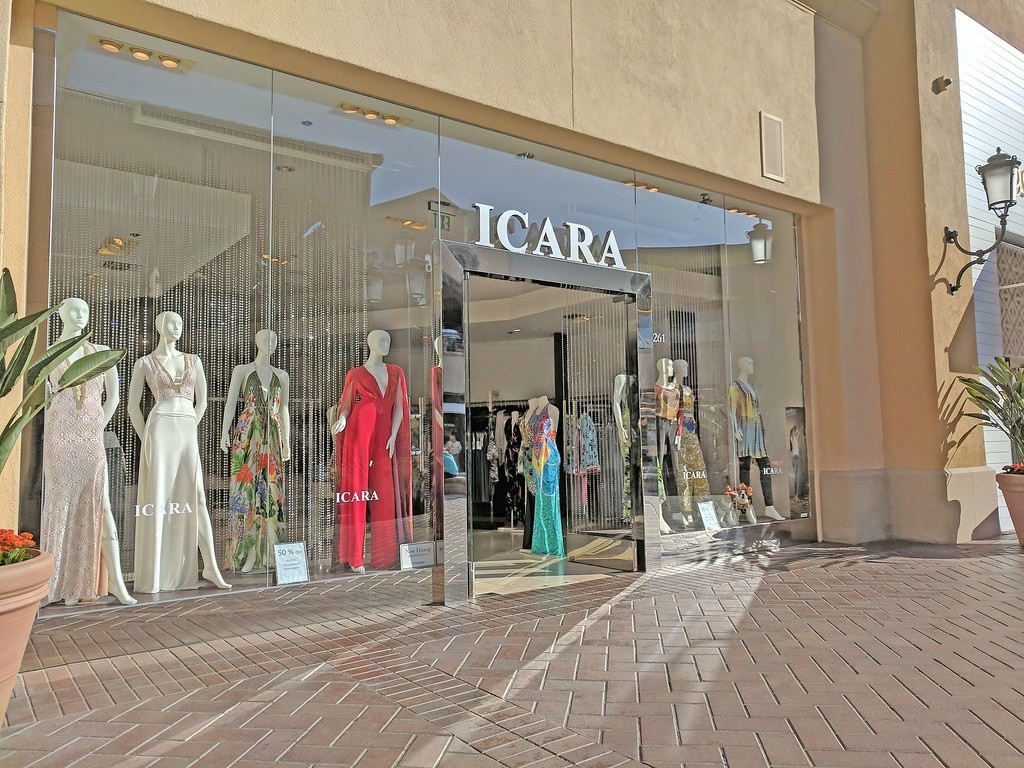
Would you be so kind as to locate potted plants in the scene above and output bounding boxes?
[0,267,127,716]
[957,355,1024,551]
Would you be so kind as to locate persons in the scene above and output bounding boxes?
[504,396,565,558]
[332,330,413,572]
[790,425,801,503]
[40,297,138,605]
[728,357,786,523]
[129,310,233,594]
[221,329,290,573]
[613,369,676,534]
[447,435,461,472]
[655,356,711,527]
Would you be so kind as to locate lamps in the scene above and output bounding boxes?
[945,147,1024,296]
[746,217,773,264]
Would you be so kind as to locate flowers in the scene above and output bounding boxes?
[0,529,37,566]
